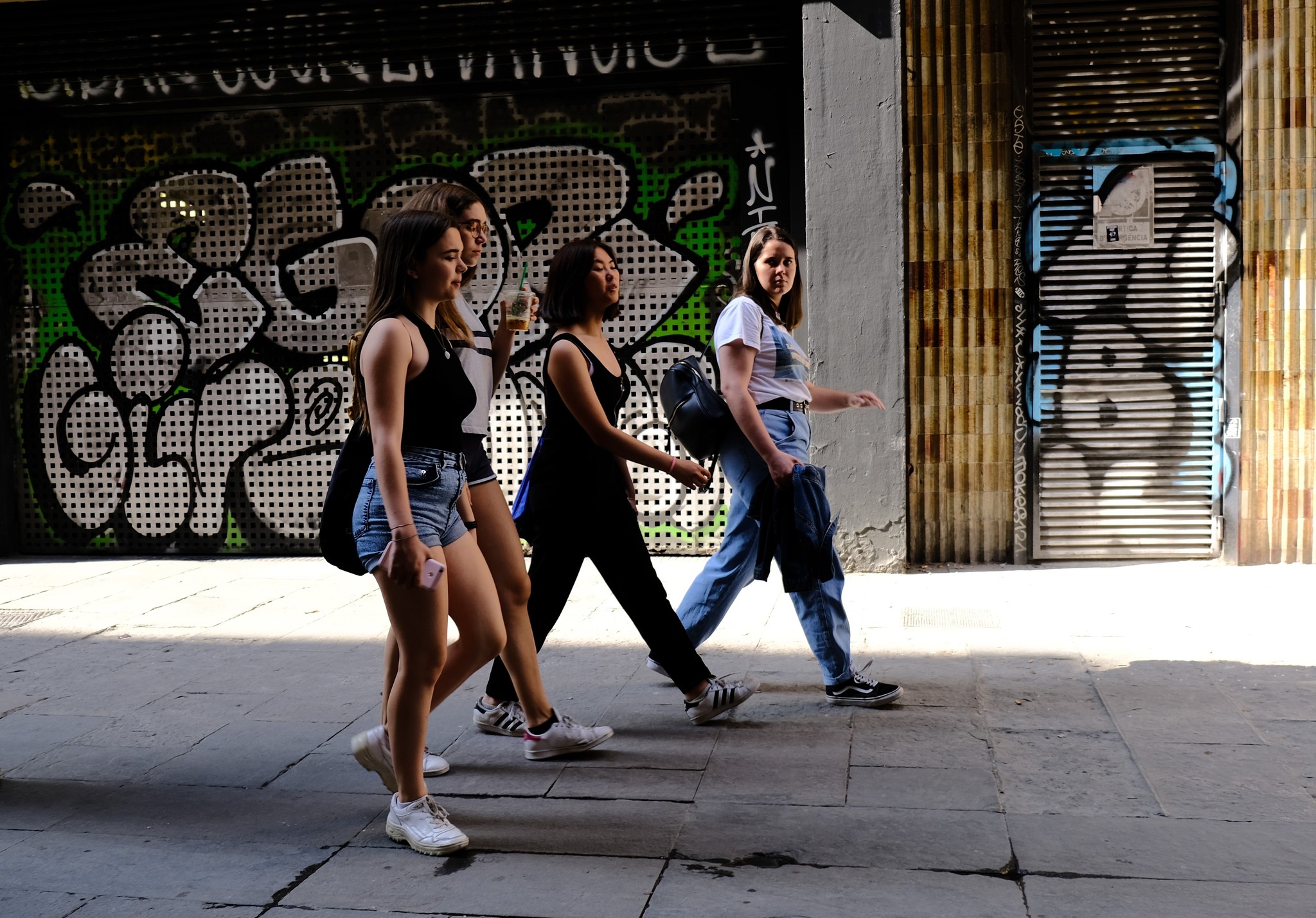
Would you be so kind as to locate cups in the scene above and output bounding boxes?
[500,290,536,331]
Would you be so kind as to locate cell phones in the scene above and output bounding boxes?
[377,541,446,592]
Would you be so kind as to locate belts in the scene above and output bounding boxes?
[756,398,810,415]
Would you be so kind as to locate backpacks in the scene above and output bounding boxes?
[658,354,743,494]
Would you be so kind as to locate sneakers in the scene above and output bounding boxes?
[523,706,614,759]
[421,746,450,777]
[645,650,717,682]
[826,657,904,707]
[472,695,528,737]
[350,725,397,794]
[385,792,469,856]
[683,671,760,725]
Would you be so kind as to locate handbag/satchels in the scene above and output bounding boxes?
[508,429,544,546]
[318,415,374,576]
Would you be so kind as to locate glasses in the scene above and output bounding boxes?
[456,221,489,239]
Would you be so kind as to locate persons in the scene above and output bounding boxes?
[352,211,509,855]
[472,239,762,736]
[646,226,904,708]
[380,184,615,776]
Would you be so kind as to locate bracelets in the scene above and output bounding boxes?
[626,496,636,500]
[665,456,677,475]
[392,533,418,541]
[462,521,478,529]
[390,523,415,531]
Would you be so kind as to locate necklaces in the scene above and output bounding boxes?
[407,306,450,359]
